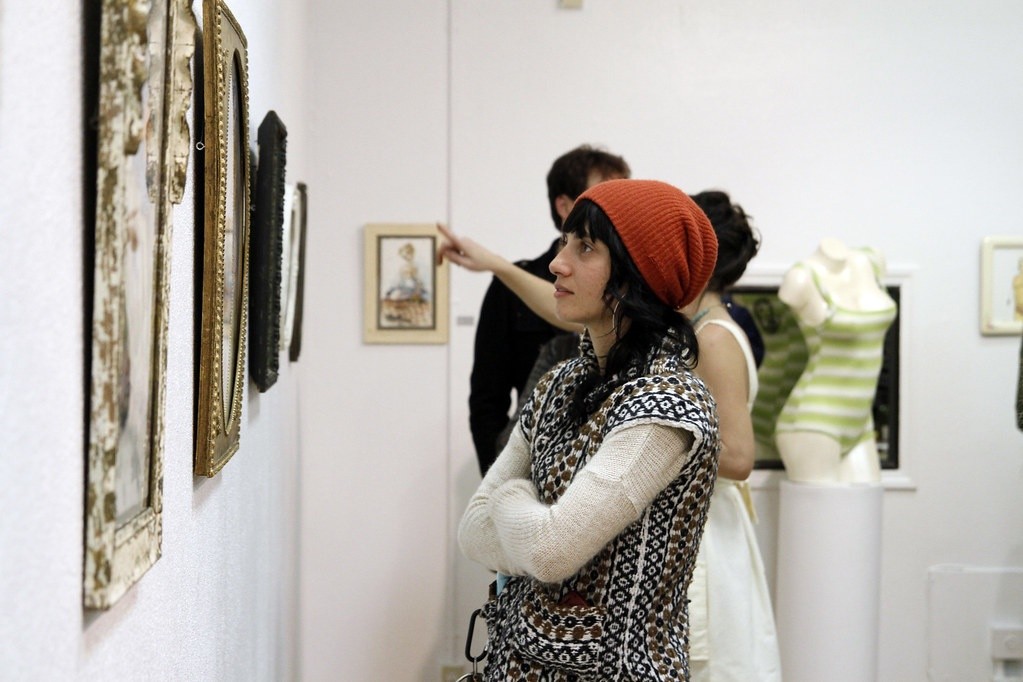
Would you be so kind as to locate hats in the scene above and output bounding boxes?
[577,179,720,310]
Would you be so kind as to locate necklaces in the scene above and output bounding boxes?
[690,299,722,327]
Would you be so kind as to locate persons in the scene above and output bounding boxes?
[467,145,635,480]
[777,243,896,484]
[457,179,721,682]
[436,189,780,682]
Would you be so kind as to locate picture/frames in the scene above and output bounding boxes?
[979,236,1023,337]
[74,0,195,611]
[362,224,451,345]
[249,106,287,392]
[724,265,920,491]
[193,0,250,478]
[287,181,306,363]
